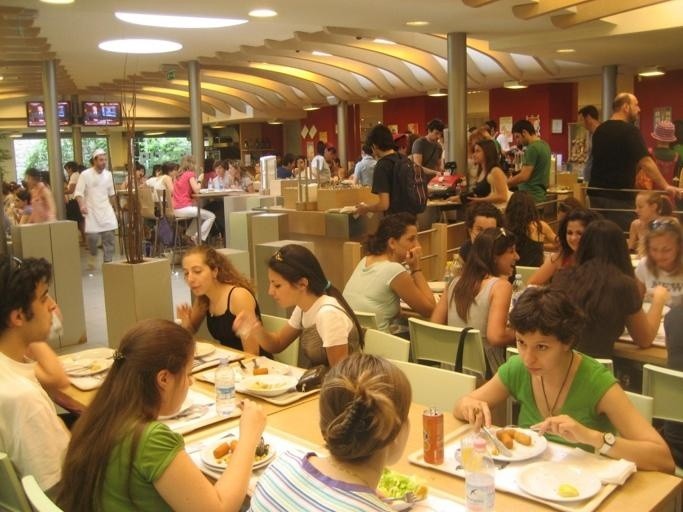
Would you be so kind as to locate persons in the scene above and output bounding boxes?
[578,92,682,229]
[2,144,336,264]
[452,286,675,476]
[173,243,365,372]
[329,117,551,228]
[53,318,266,512]
[342,190,681,396]
[0,255,73,512]
[243,352,413,512]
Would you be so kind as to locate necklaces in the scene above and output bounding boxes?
[540,350,573,417]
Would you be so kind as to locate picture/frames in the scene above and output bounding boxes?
[551,118,563,134]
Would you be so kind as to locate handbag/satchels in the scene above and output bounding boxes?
[636,167,653,190]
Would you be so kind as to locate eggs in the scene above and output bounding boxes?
[555,483,581,498]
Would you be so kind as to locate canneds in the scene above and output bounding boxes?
[423,407,444,464]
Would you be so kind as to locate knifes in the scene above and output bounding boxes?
[482,425,513,458]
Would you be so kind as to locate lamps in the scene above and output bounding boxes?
[503,80,529,90]
[209,88,447,130]
[637,66,668,76]
[7,128,167,140]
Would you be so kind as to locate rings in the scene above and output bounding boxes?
[473,407,480,415]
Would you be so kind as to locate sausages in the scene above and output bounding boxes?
[230,439,237,453]
[253,368,266,374]
[214,440,230,457]
[496,426,533,449]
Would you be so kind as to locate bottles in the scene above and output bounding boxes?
[511,274,524,308]
[208,177,214,191]
[215,358,235,415]
[465,439,496,512]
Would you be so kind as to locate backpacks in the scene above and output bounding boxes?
[379,152,426,214]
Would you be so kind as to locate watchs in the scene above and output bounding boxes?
[597,433,615,456]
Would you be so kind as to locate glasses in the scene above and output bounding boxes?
[7,252,22,291]
[649,220,675,230]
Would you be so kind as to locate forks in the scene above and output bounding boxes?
[379,492,417,503]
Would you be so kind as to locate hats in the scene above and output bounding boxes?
[92,148,106,158]
[323,142,335,150]
[392,133,408,146]
[650,121,677,142]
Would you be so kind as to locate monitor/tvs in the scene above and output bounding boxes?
[81,101,122,126]
[25,101,72,127]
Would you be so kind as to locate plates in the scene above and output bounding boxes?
[516,462,601,502]
[65,359,114,376]
[201,438,277,472]
[192,342,216,358]
[462,429,548,461]
[239,375,298,396]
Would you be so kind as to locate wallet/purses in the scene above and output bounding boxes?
[296,364,327,391]
